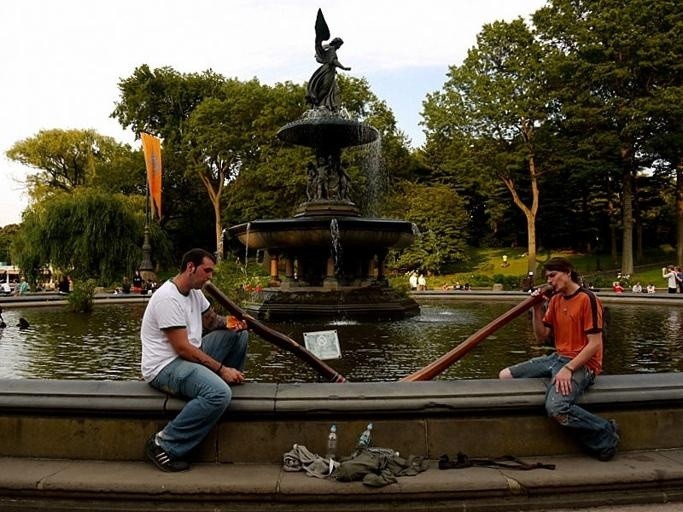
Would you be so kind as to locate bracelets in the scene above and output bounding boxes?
[215,362,222,374]
[563,365,574,372]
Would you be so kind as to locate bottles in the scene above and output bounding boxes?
[356,422,374,451]
[327,424,339,463]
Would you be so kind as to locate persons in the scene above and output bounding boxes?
[521,271,535,293]
[442,281,472,293]
[305,35,351,110]
[408,271,417,291]
[645,283,655,294]
[671,265,682,294]
[612,282,624,294]
[631,281,642,293]
[660,265,677,293]
[0,270,158,299]
[139,248,250,473]
[497,254,622,463]
[417,273,427,291]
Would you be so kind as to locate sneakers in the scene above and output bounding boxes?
[143,433,189,472]
[598,419,620,462]
